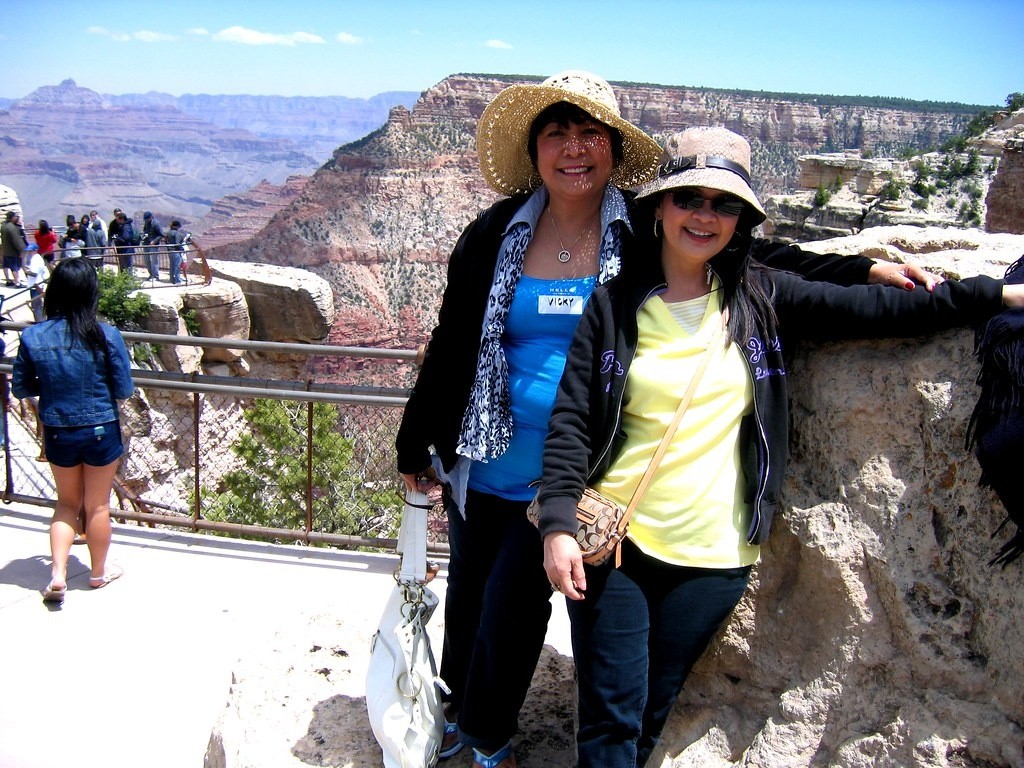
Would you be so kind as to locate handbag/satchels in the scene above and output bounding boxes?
[526,482,631,569]
[365,488,444,768]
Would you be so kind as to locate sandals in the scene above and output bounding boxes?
[43,576,68,602]
[89,563,123,589]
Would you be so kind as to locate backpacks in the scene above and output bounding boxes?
[131,230,141,246]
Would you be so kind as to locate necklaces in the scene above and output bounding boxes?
[547,207,599,263]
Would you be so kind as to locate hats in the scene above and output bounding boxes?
[172,221,182,227]
[25,243,39,251]
[631,126,767,232]
[476,70,665,198]
[143,212,152,221]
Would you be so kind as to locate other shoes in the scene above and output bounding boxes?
[145,276,159,281]
[6,280,28,288]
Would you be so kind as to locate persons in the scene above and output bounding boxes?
[12,256,135,603]
[395,70,947,767]
[532,125,1024,768]
[0,208,190,323]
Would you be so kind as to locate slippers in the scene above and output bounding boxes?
[437,709,514,768]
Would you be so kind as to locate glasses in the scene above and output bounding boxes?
[395,471,452,513]
[666,187,746,217]
